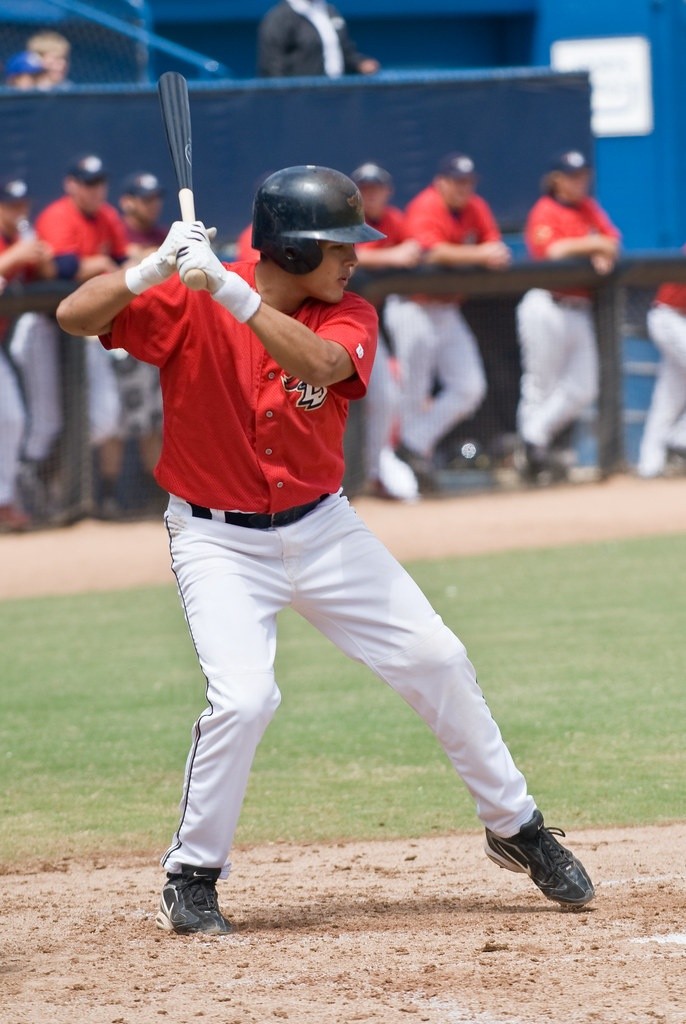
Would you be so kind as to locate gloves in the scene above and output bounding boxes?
[125,221,218,295]
[175,241,262,324]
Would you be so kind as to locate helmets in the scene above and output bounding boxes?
[250,165,387,274]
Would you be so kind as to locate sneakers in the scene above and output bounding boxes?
[484,809,594,907]
[157,865,233,935]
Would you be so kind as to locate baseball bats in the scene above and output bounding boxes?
[158,71,208,290]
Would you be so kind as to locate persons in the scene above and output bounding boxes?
[0,154,170,527]
[236,223,261,262]
[635,285,686,478]
[379,154,512,497]
[491,149,620,492]
[260,0,379,80]
[0,30,73,93]
[57,165,595,934]
[339,162,423,498]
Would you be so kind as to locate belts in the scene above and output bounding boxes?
[187,491,329,530]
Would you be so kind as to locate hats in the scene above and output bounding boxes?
[439,151,481,179]
[548,146,592,173]
[351,163,392,187]
[66,152,109,184]
[0,177,31,201]
[117,171,165,200]
